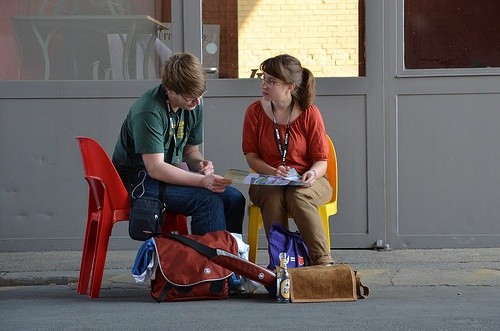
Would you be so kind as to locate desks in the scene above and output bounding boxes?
[14,15,170,80]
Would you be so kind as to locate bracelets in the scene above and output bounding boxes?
[311,168,318,179]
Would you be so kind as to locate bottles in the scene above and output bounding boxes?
[277,253,291,303]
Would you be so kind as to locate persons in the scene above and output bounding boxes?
[242,54,336,267]
[112,51,246,236]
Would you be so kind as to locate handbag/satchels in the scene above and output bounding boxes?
[261,223,311,293]
[274,263,370,304]
[129,197,166,241]
[151,231,239,301]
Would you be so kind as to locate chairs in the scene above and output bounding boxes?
[75,136,188,299]
[248,133,338,264]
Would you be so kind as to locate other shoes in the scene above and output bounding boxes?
[230,285,245,294]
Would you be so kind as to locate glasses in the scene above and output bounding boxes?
[263,76,293,85]
[173,87,208,105]
